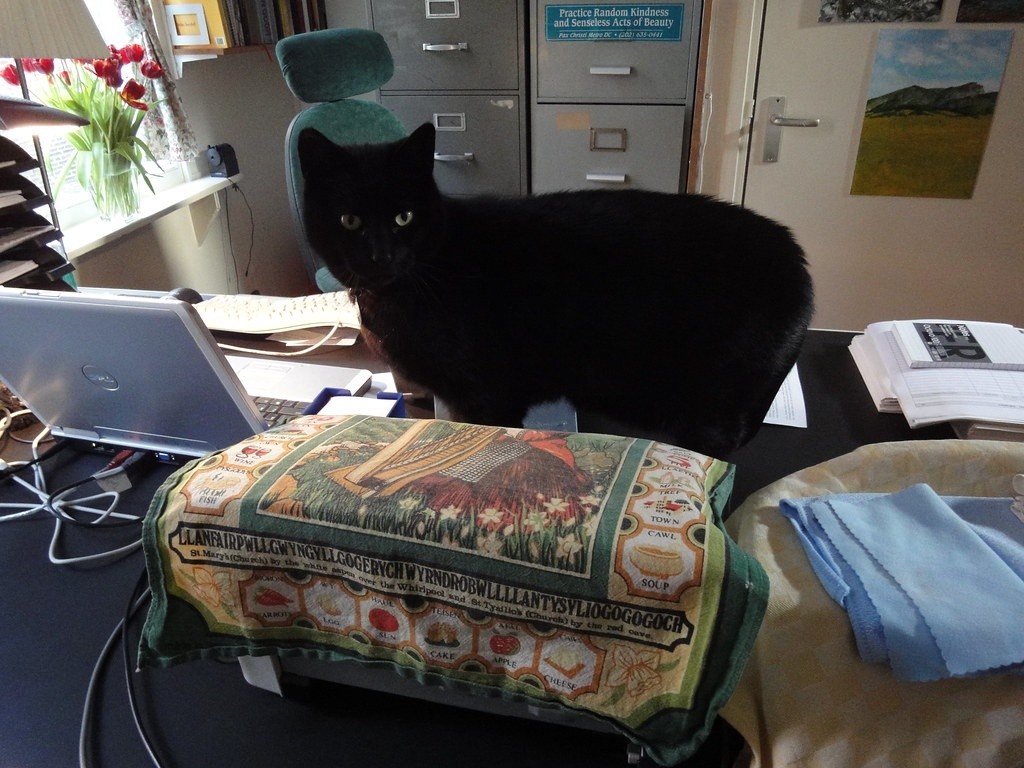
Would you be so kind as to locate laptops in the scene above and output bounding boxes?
[0,286,373,467]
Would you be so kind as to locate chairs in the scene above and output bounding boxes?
[275,27,410,294]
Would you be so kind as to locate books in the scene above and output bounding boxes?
[163,0,328,49]
[849,319,1024,429]
[0,161,55,283]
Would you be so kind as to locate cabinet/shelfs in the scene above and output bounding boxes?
[0,135,77,292]
[530,0,702,198]
[366,0,530,196]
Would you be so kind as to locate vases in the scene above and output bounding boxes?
[88,146,141,224]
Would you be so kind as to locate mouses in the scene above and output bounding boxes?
[161,287,204,305]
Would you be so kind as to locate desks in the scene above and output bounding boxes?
[0,287,958,768]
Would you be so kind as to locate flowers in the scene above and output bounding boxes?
[0,44,169,216]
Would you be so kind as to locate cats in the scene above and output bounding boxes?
[297,121,816,462]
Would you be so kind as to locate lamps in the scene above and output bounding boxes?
[0,95,90,137]
[0,0,111,263]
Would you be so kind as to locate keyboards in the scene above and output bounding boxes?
[191,292,361,335]
